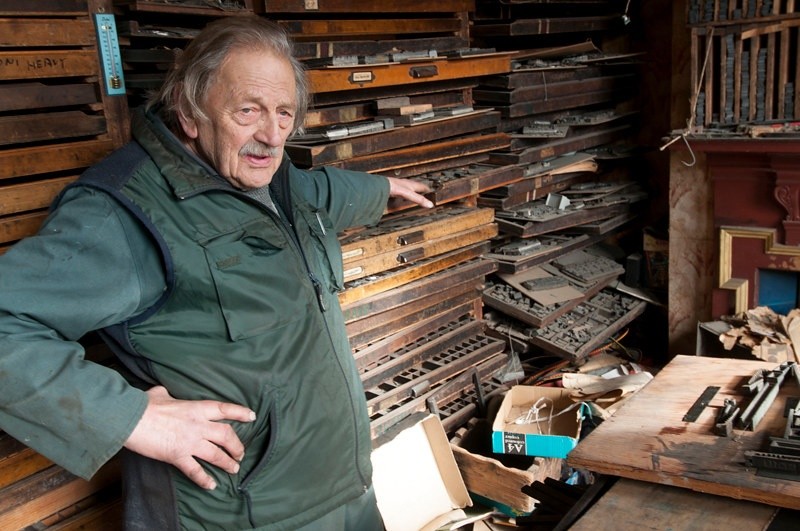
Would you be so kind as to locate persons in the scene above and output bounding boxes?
[0,11,437,531]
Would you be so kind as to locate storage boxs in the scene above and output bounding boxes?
[370,411,474,531]
[492,385,582,459]
[450,419,561,518]
